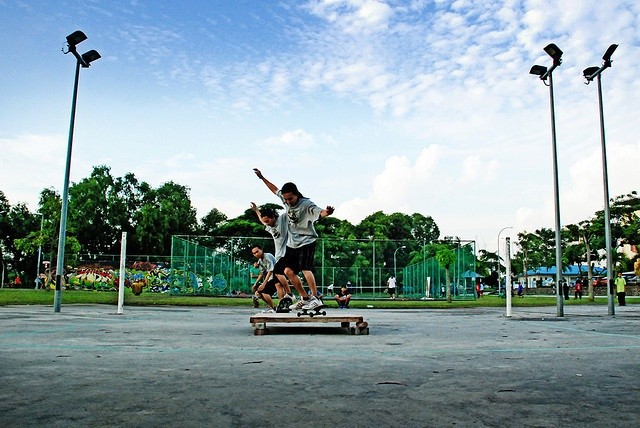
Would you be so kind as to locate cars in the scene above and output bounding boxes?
[600,278,607,286]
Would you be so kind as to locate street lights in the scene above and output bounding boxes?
[34,212,43,289]
[394,246,406,293]
[530,43,563,317]
[54,30,101,311]
[583,44,618,315]
[498,227,513,293]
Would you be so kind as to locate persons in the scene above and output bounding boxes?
[307,285,324,304]
[614,270,627,306]
[582,277,588,287]
[346,280,353,294]
[479,281,484,298]
[475,281,480,298]
[517,282,524,298]
[548,279,556,295]
[250,201,296,301]
[15,275,21,288]
[334,285,352,310]
[327,283,334,297]
[253,167,335,311]
[573,279,583,300]
[250,243,277,313]
[562,279,569,300]
[386,274,398,300]
[249,260,264,309]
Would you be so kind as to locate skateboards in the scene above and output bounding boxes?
[295,304,327,317]
[252,296,259,307]
[276,297,293,312]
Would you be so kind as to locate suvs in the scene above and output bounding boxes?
[592,275,602,287]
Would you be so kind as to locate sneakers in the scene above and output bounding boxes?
[301,294,323,309]
[289,295,310,310]
[284,292,296,301]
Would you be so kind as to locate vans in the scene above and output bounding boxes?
[622,272,637,286]
